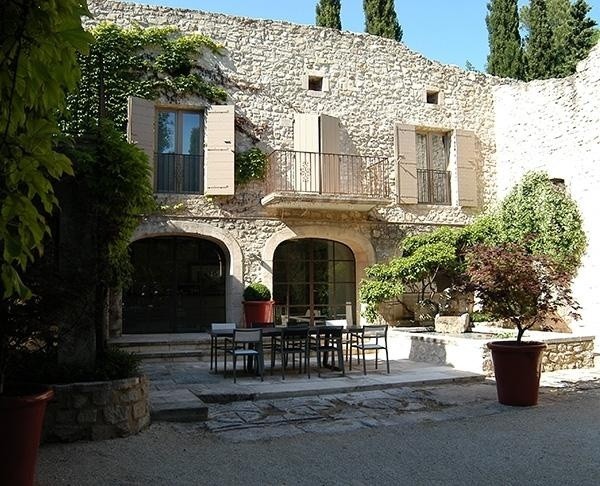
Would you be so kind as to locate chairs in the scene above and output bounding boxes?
[206,319,390,383]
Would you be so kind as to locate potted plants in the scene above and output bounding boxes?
[0,289,96,486]
[458,241,557,406]
[241,282,276,328]
